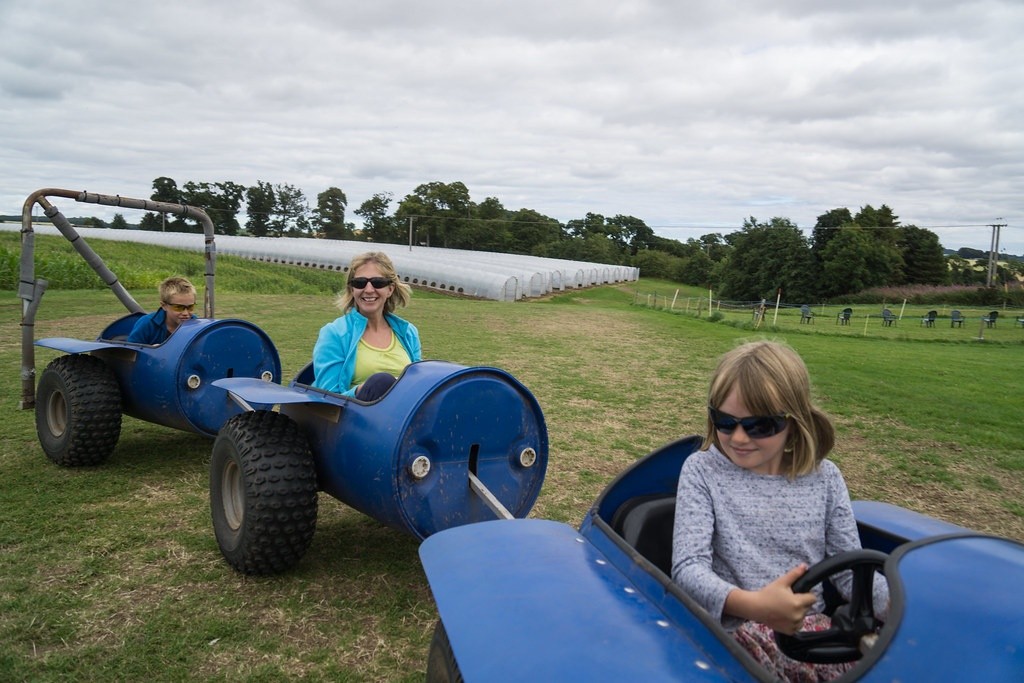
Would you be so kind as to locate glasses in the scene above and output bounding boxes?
[348,277,393,290]
[707,406,792,441]
[163,301,195,313]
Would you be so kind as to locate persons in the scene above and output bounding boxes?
[127,276,199,346]
[310,251,422,401]
[671,341,890,683]
